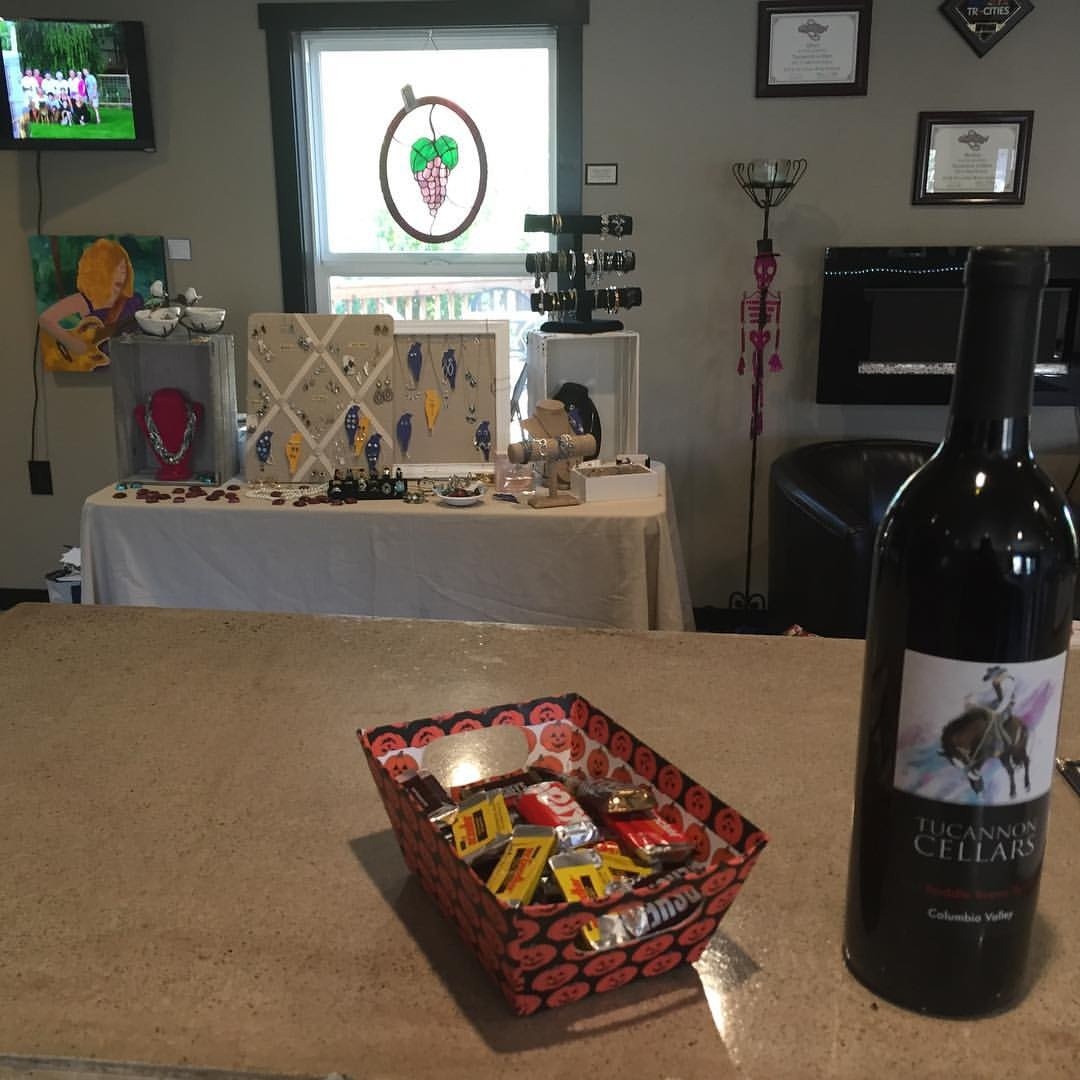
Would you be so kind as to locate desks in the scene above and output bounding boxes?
[0,603,1079,1079]
[81,459,697,632]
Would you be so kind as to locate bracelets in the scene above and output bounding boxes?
[533,214,632,319]
[516,434,575,465]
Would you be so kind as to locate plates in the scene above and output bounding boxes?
[436,490,488,507]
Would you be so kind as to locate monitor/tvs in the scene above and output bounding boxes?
[815,244,1080,407]
[0,17,159,153]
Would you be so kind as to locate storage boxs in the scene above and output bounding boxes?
[108,332,236,487]
[356,691,769,1019]
[526,330,639,461]
[569,463,659,502]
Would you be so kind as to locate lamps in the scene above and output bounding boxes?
[729,157,809,611]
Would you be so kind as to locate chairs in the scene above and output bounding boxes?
[768,439,941,641]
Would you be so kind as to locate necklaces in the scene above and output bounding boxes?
[145,389,195,465]
[396,318,497,425]
[566,396,596,434]
[533,414,576,468]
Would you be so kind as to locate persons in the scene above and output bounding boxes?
[21,69,100,124]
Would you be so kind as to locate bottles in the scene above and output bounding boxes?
[843,247,1079,1016]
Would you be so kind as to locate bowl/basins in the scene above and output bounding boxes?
[134,307,181,336]
[186,306,227,331]
